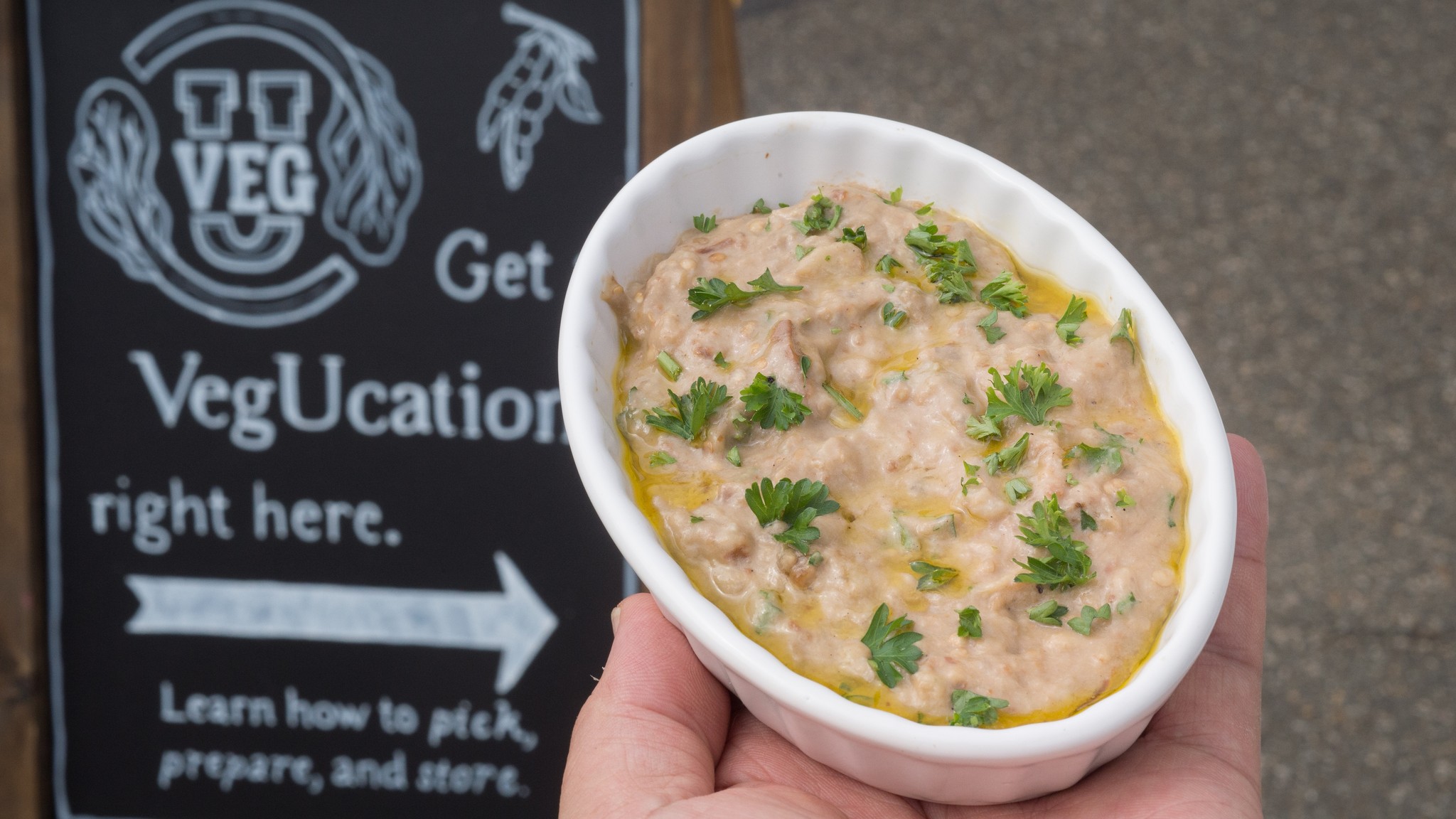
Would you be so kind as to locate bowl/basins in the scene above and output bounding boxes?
[557,110,1237,807]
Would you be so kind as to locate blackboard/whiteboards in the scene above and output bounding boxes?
[0,0,742,818]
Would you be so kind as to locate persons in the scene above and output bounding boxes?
[558,433,1269,819]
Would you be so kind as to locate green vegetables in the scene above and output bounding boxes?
[644,185,1177,729]
